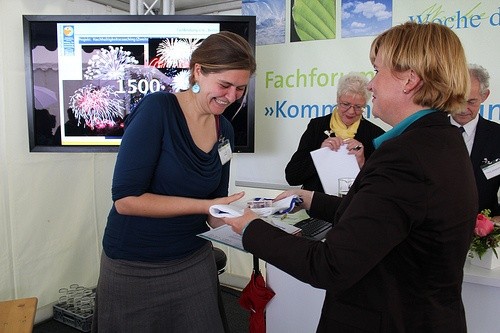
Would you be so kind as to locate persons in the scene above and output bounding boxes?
[284,75,388,195]
[32,106,96,146]
[447,62,500,218]
[91,31,258,333]
[222,19,481,333]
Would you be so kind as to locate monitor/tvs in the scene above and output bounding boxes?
[23,14,255,155]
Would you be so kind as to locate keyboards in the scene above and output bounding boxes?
[293,217,333,240]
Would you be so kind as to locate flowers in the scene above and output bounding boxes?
[468,208,500,259]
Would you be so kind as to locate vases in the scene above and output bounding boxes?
[470,242,500,270]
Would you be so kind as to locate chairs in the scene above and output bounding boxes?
[213,247,230,333]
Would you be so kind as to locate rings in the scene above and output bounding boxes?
[355,145,362,150]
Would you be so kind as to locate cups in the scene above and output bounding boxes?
[57,283,95,317]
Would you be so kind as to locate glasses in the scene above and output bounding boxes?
[339,101,365,111]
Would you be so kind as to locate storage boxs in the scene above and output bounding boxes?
[53,287,97,333]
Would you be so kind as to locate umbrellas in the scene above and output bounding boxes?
[34,85,57,109]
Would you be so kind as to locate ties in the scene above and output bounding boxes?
[459,126,465,134]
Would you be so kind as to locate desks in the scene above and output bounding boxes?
[266,250,500,333]
[0,297,38,333]
